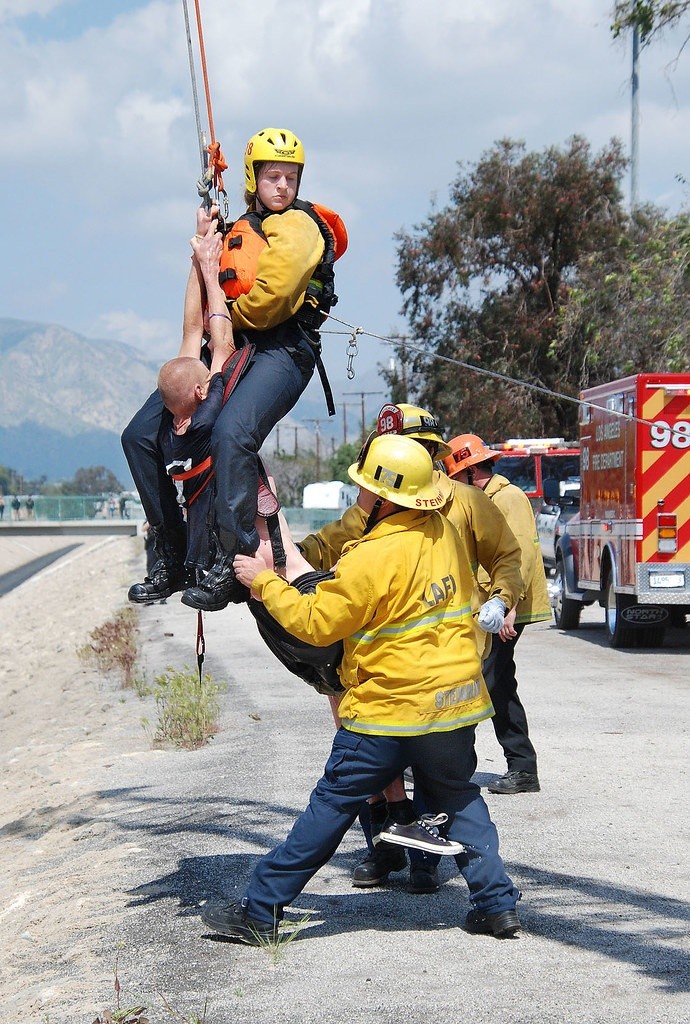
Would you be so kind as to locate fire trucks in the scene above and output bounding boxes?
[489,438,581,515]
[541,373,690,649]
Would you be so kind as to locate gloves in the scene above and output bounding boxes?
[476,597,507,634]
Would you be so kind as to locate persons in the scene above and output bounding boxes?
[12,495,20,521]
[201,434,522,943]
[122,127,348,612]
[158,219,464,856]
[440,434,550,792]
[26,494,35,519]
[109,496,130,520]
[292,402,525,892]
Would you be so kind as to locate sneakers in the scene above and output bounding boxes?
[201,900,279,946]
[409,855,440,893]
[128,557,196,601]
[182,562,254,612]
[352,843,407,886]
[371,823,397,849]
[487,770,539,793]
[465,902,521,936]
[379,815,464,855]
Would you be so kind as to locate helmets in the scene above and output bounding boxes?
[347,432,447,509]
[440,434,502,475]
[381,401,454,461]
[244,128,306,201]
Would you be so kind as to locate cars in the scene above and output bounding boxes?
[535,475,582,577]
